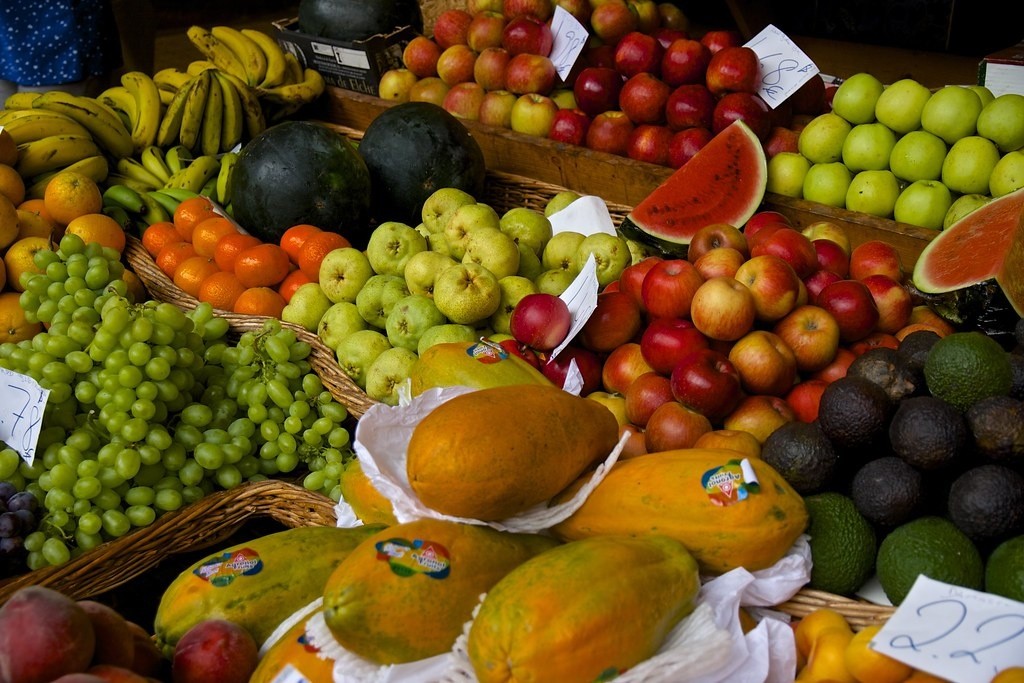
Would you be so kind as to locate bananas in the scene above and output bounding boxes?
[0,25,328,242]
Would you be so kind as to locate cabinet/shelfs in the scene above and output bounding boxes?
[271,0,1024,276]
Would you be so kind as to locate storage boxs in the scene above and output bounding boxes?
[1,473,901,682]
[975,41,1024,100]
[116,119,643,416]
[272,16,435,98]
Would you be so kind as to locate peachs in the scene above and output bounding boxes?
[0,581,258,683]
[738,605,1023,683]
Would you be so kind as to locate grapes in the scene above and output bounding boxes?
[0,234,357,585]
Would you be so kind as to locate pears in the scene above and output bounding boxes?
[282,186,659,409]
[770,72,1023,232]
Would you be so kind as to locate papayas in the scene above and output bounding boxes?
[154,341,808,683]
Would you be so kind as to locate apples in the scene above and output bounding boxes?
[378,0,840,168]
[495,212,956,460]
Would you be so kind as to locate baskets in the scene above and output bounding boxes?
[1,120,904,632]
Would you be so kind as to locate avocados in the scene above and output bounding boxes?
[759,330,1024,607]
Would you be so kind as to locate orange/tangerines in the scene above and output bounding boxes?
[0,126,151,346]
[141,198,352,324]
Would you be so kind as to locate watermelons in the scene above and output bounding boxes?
[617,119,768,261]
[910,188,1024,351]
[229,103,489,246]
[298,0,424,40]
